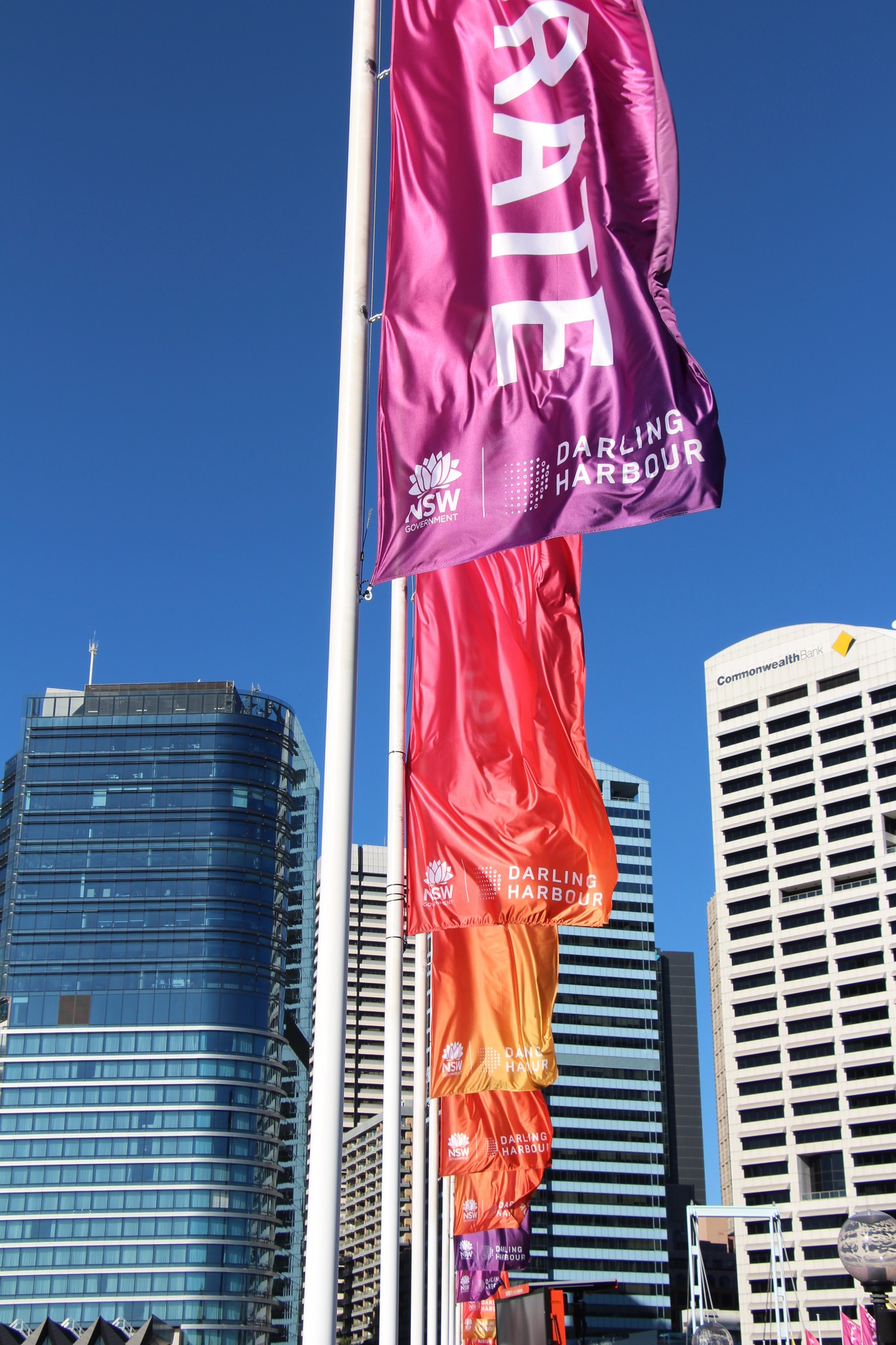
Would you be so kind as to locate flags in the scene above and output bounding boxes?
[453,1168,545,1237]
[406,534,618,935]
[440,1090,554,1177]
[368,0,727,586]
[429,925,560,1101]
[456,1270,506,1303]
[462,1271,510,1345]
[859,1304,878,1345]
[841,1312,862,1345]
[885,1298,896,1310]
[805,1328,820,1345]
[453,1205,532,1272]
